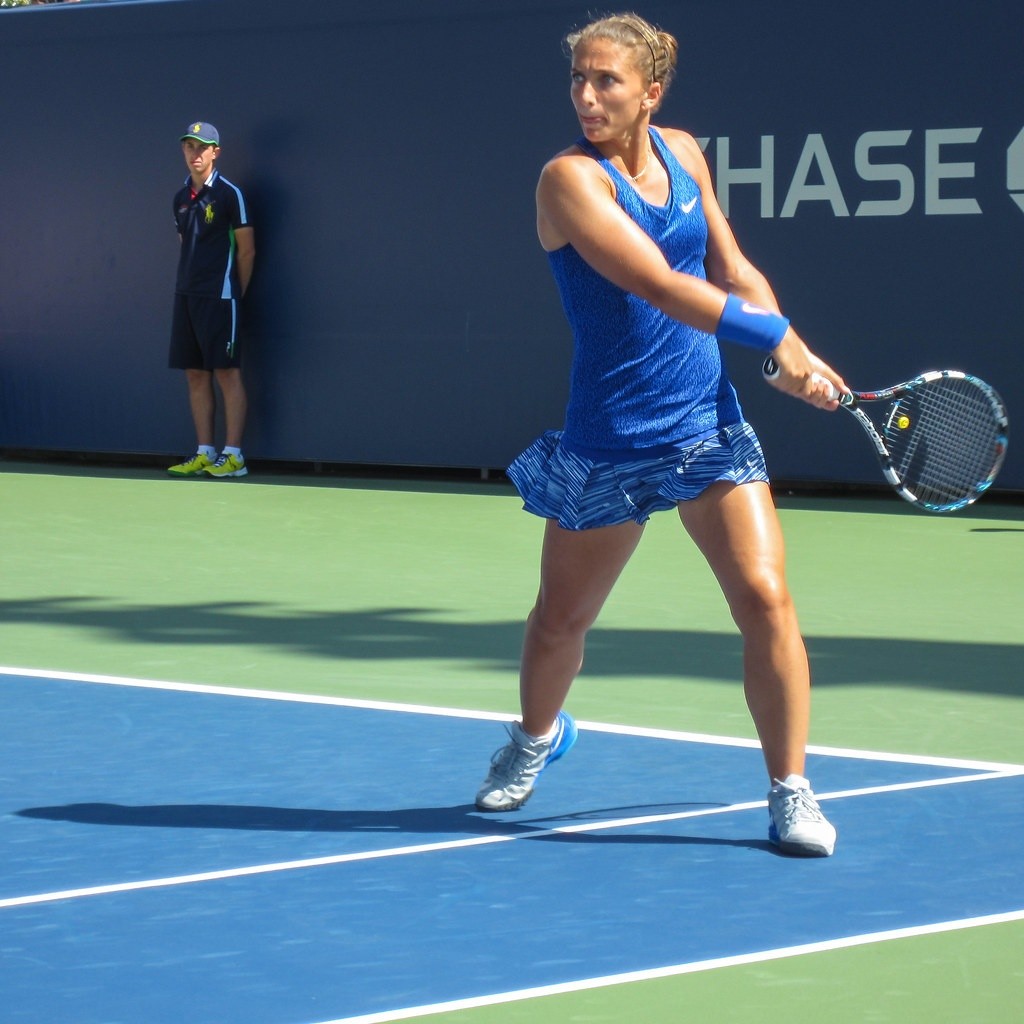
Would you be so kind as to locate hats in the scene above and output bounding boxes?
[178,122,220,146]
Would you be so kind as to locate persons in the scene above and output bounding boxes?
[473,12,853,859]
[162,119,257,480]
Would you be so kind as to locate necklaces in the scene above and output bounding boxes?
[618,132,651,182]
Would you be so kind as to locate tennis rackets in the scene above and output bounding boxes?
[761,355,1010,514]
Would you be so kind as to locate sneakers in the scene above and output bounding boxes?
[202,450,248,477]
[167,451,219,478]
[768,772,836,857]
[474,709,578,810]
